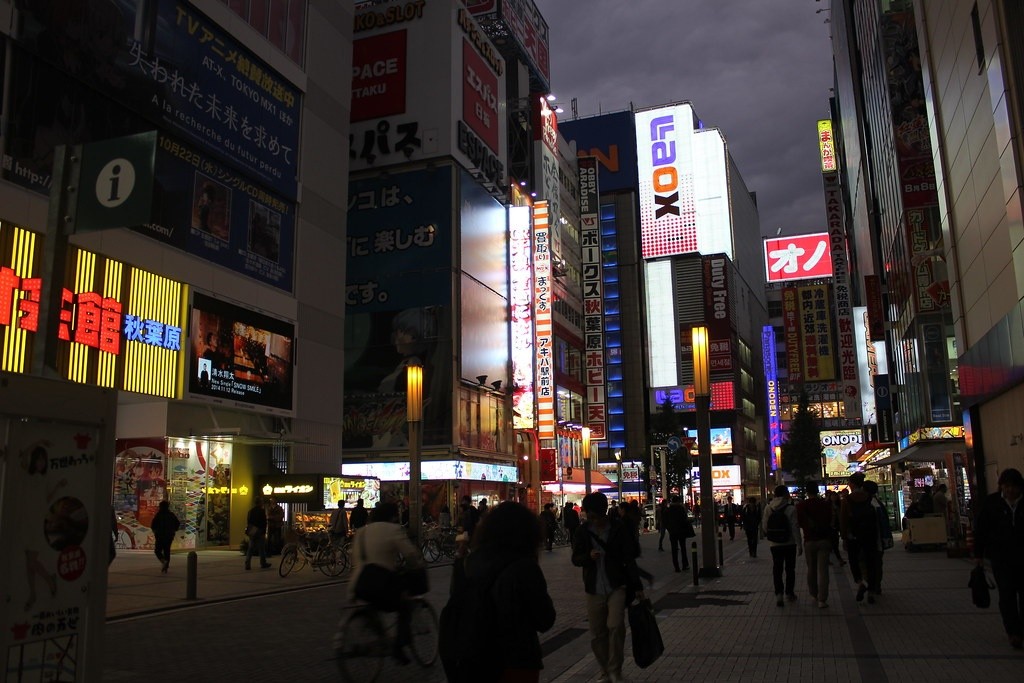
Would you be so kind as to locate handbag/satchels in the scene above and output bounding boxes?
[581,521,644,591]
[245,510,266,541]
[968,561,996,608]
[352,527,404,613]
[332,530,347,538]
[628,597,667,669]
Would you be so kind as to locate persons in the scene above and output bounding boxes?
[243,496,272,570]
[968,466,1024,653]
[571,491,641,683]
[324,471,974,613]
[449,500,557,682]
[150,500,180,573]
[267,495,286,558]
[108,502,118,568]
[344,500,426,668]
[185,175,293,409]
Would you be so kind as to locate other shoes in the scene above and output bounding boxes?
[261,563,272,569]
[675,568,681,572]
[828,562,834,565]
[161,560,171,574]
[245,567,251,570]
[785,590,798,601]
[812,597,818,601]
[818,602,829,608]
[777,601,785,607]
[682,566,690,570]
[544,546,552,552]
[840,560,847,567]
[867,595,877,605]
[856,583,866,602]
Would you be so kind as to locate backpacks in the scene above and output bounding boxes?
[437,551,537,683]
[765,501,792,543]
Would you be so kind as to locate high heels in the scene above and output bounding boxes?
[659,546,665,552]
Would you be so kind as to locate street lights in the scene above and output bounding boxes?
[405,358,425,551]
[582,425,593,498]
[614,448,622,503]
[690,321,725,577]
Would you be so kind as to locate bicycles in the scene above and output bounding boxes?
[421,525,468,562]
[548,521,570,545]
[275,513,355,578]
[324,555,442,683]
